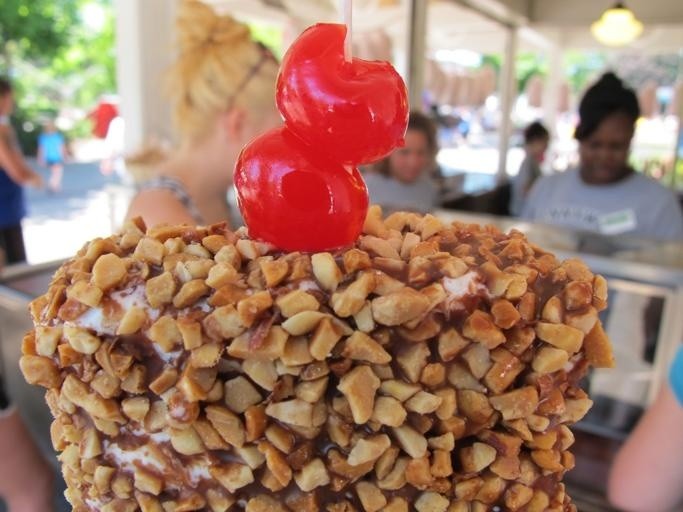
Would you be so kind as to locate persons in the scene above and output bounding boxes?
[0,76,42,266]
[608,344,683,512]
[0,379,57,512]
[37,108,66,169]
[519,72,683,245]
[507,123,549,216]
[379,111,438,185]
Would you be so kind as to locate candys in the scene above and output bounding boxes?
[233,0,409,254]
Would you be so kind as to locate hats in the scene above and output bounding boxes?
[576,73,621,139]
